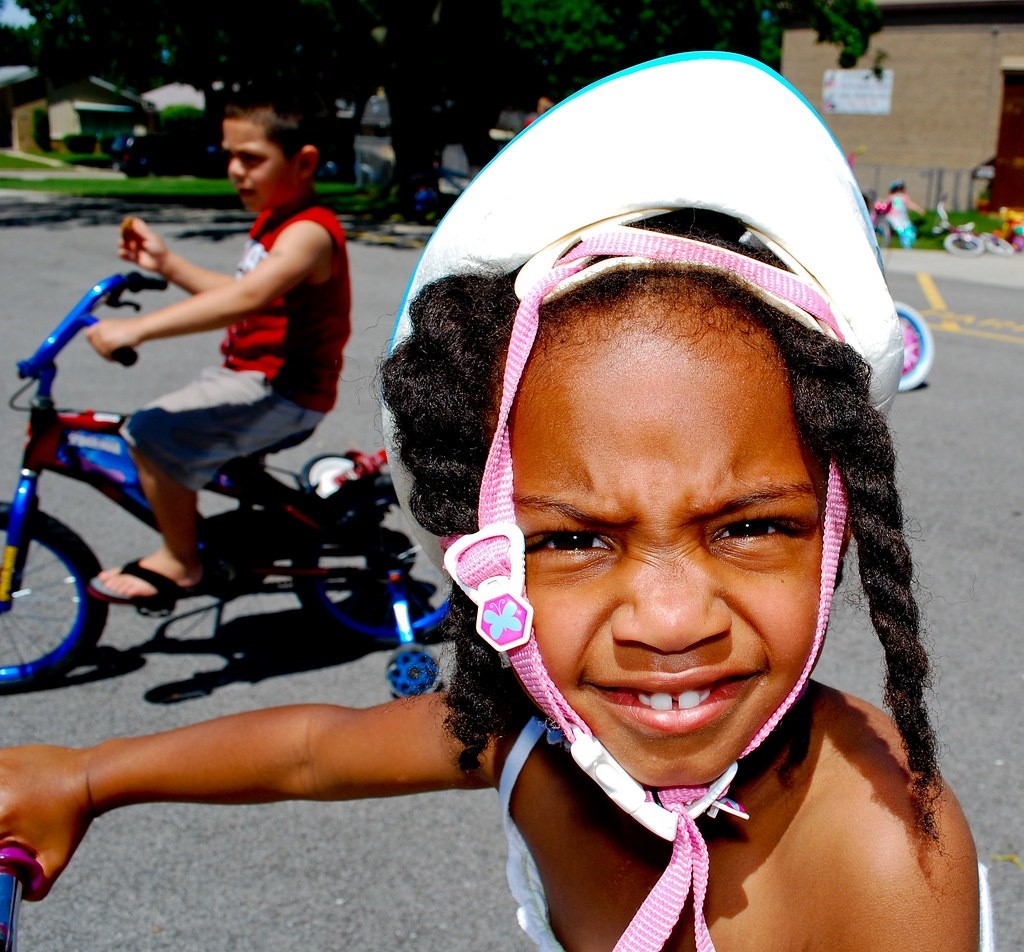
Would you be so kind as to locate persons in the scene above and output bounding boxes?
[0,52,996,952]
[869,181,928,248]
[84,97,356,603]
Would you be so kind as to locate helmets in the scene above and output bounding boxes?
[381,50,905,596]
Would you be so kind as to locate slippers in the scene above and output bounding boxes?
[88,557,218,602]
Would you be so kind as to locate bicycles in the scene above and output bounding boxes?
[887,300,934,393]
[929,194,1014,257]
[0,271,456,696]
